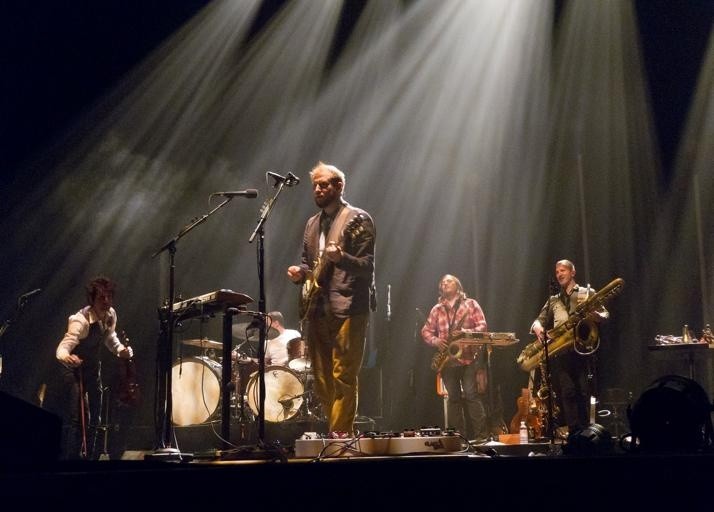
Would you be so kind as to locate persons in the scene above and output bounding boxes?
[530,259,609,431]
[56,278,133,429]
[288,162,375,438]
[422,273,487,436]
[259,310,301,367]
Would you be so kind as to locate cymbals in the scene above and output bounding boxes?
[181,338,223,350]
[231,321,280,341]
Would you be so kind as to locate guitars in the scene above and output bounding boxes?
[511,368,542,439]
[112,329,139,411]
[295,214,365,321]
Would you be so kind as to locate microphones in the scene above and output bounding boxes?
[386,284,391,323]
[22,288,42,299]
[269,171,293,187]
[214,189,257,198]
[289,172,300,185]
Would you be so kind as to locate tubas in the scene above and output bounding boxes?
[517,278,626,372]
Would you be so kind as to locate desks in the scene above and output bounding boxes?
[646,340,714,382]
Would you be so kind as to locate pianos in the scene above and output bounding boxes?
[163,289,253,310]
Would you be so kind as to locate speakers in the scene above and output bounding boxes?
[0,391,63,462]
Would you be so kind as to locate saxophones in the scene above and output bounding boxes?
[431,299,470,372]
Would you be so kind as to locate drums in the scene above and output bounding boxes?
[287,337,313,371]
[165,355,224,426]
[245,365,306,423]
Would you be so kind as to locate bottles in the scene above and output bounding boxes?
[519,421,529,444]
[705,323,712,343]
[683,324,691,344]
